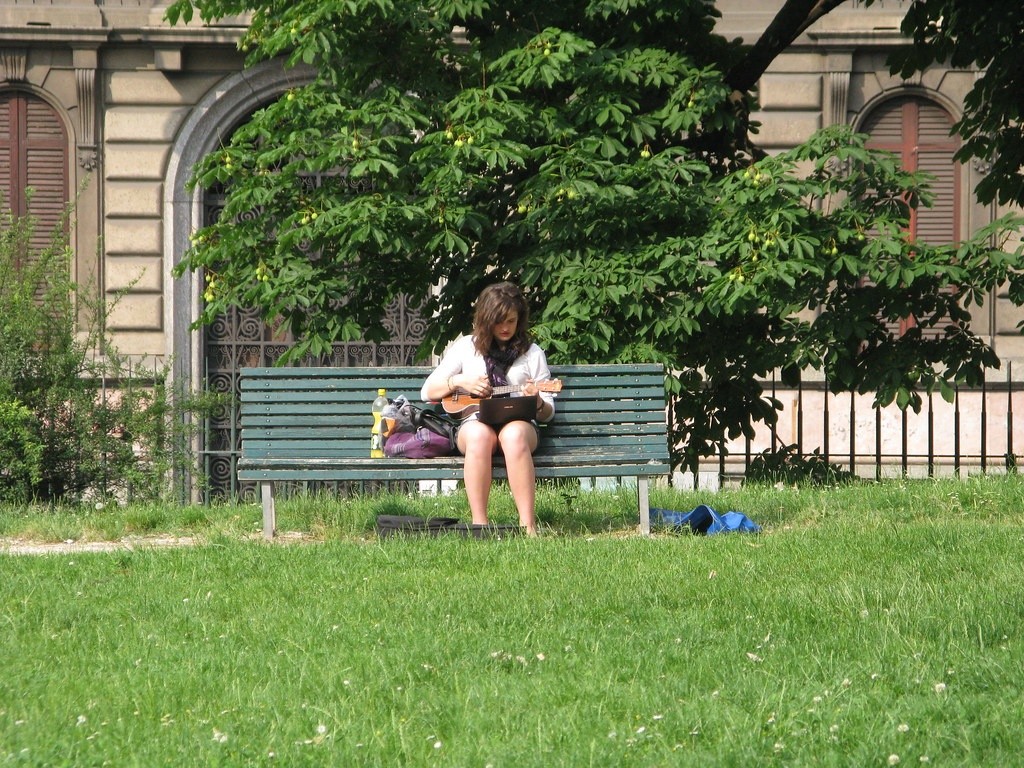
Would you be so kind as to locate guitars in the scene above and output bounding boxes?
[441,376,562,421]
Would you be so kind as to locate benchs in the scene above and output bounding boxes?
[237,362,672,539]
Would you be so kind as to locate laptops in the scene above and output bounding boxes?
[476,395,538,425]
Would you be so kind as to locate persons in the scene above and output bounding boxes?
[421,283,555,537]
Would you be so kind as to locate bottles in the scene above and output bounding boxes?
[370,389,389,458]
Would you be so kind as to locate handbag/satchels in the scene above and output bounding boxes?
[384,408,456,458]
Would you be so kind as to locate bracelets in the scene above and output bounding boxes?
[537,400,545,412]
[447,376,458,391]
